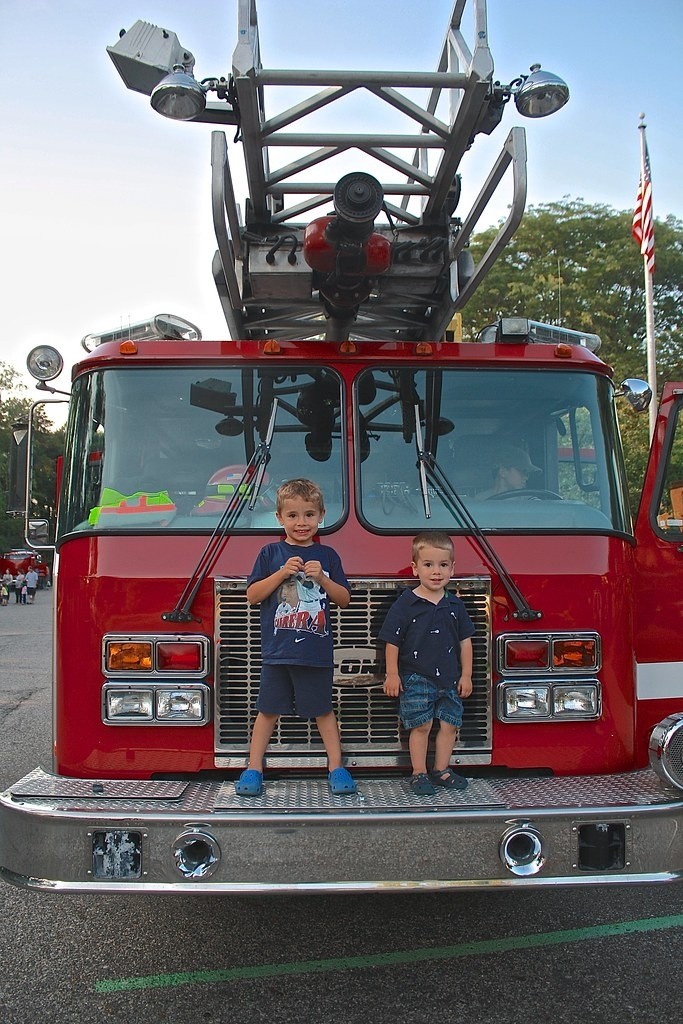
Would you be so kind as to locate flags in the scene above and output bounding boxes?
[632,144,655,273]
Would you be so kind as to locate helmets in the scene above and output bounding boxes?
[191,465,280,516]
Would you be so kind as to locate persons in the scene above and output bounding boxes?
[377,532,476,794]
[235,478,357,795]
[3,568,13,600]
[474,446,542,500]
[24,566,38,604]
[15,570,24,603]
[21,582,27,605]
[1,582,8,606]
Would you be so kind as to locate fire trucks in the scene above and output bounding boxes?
[0,0,683,890]
[0,547,47,588]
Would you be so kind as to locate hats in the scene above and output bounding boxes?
[495,447,543,476]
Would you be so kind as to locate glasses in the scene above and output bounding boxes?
[511,465,530,478]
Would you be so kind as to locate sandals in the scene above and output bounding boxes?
[235,770,263,796]
[327,767,356,794]
[429,765,468,789]
[409,772,436,795]
